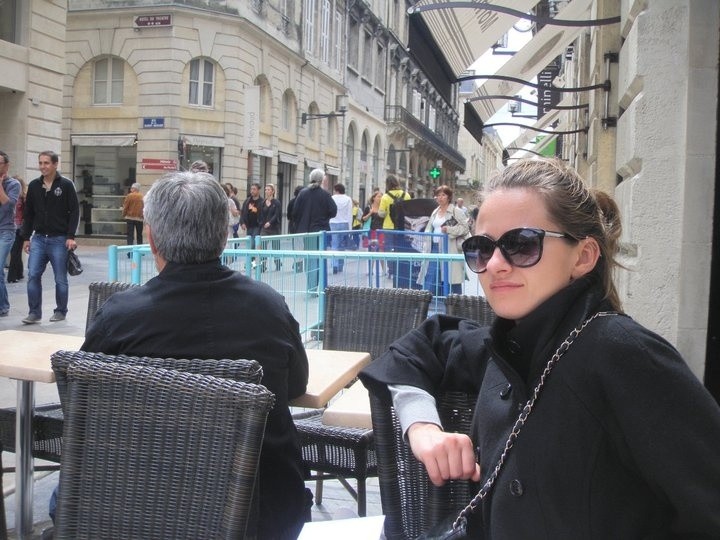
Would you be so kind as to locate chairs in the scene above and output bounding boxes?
[0,286,502,539]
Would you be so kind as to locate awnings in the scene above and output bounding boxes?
[407,0,593,167]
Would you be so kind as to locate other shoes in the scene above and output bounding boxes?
[333,266,339,274]
[274,259,284,271]
[260,260,268,273]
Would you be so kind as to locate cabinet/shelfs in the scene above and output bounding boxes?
[90,194,127,233]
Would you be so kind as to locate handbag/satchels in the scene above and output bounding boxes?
[66,250,84,277]
[360,216,373,237]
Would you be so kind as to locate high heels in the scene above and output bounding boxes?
[7,272,26,284]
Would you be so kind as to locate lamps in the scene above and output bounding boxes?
[302,91,350,123]
[382,135,416,156]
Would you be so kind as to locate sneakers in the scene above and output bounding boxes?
[50,311,67,321]
[22,311,43,324]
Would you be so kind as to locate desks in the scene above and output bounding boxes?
[0,328,371,539]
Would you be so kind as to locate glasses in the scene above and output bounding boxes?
[462,226,566,274]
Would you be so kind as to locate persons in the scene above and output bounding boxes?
[357,154,720,540]
[220,169,482,296]
[49,171,314,540]
[122,183,144,259]
[190,160,208,172]
[0,150,79,324]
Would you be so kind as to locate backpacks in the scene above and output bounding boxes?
[386,189,405,224]
[452,205,473,253]
